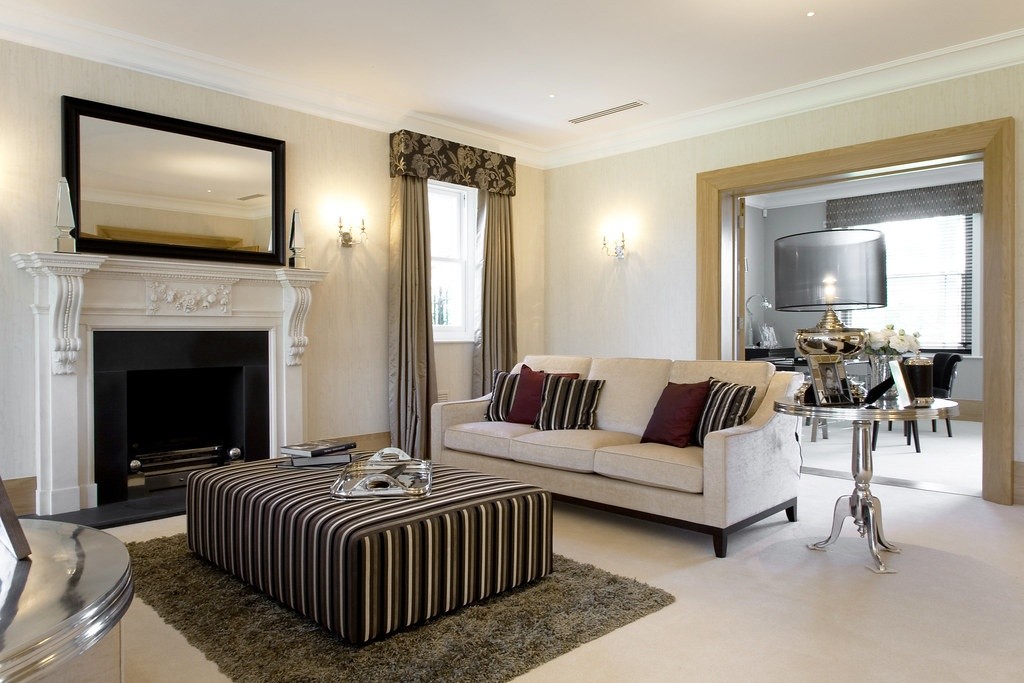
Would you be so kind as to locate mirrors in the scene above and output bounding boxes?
[59,95,287,267]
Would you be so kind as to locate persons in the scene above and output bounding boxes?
[820,366,841,394]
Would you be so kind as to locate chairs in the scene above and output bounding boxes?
[871,353,962,452]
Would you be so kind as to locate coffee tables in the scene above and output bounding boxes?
[186,449,553,646]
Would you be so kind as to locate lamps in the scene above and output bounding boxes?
[337,217,366,248]
[775,229,887,406]
[602,231,626,260]
[746,294,772,346]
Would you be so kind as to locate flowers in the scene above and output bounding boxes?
[860,325,921,357]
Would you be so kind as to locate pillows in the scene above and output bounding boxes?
[505,364,580,424]
[483,369,545,422]
[640,379,712,448]
[530,372,606,431]
[689,376,757,448]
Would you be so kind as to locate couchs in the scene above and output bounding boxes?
[430,355,805,559]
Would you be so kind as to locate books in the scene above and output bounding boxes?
[276,439,357,470]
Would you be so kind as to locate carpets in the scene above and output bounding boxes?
[122,532,677,683]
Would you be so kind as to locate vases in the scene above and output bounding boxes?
[866,355,902,408]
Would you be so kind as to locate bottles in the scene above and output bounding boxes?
[902,351,934,408]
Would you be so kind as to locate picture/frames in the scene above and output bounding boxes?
[805,353,854,406]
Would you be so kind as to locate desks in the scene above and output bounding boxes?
[745,347,797,372]
[774,397,960,574]
[0,519,135,683]
